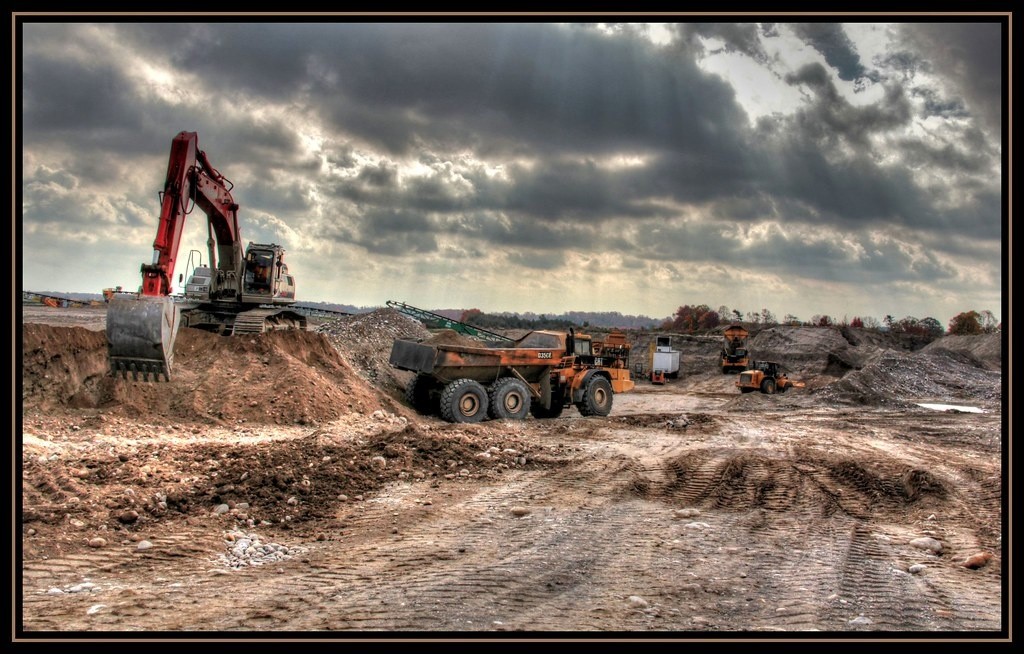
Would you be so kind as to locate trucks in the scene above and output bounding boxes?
[389,326,635,424]
[719,325,750,375]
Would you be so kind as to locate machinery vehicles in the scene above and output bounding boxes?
[107,130,307,382]
[91,288,116,306]
[735,361,805,395]
